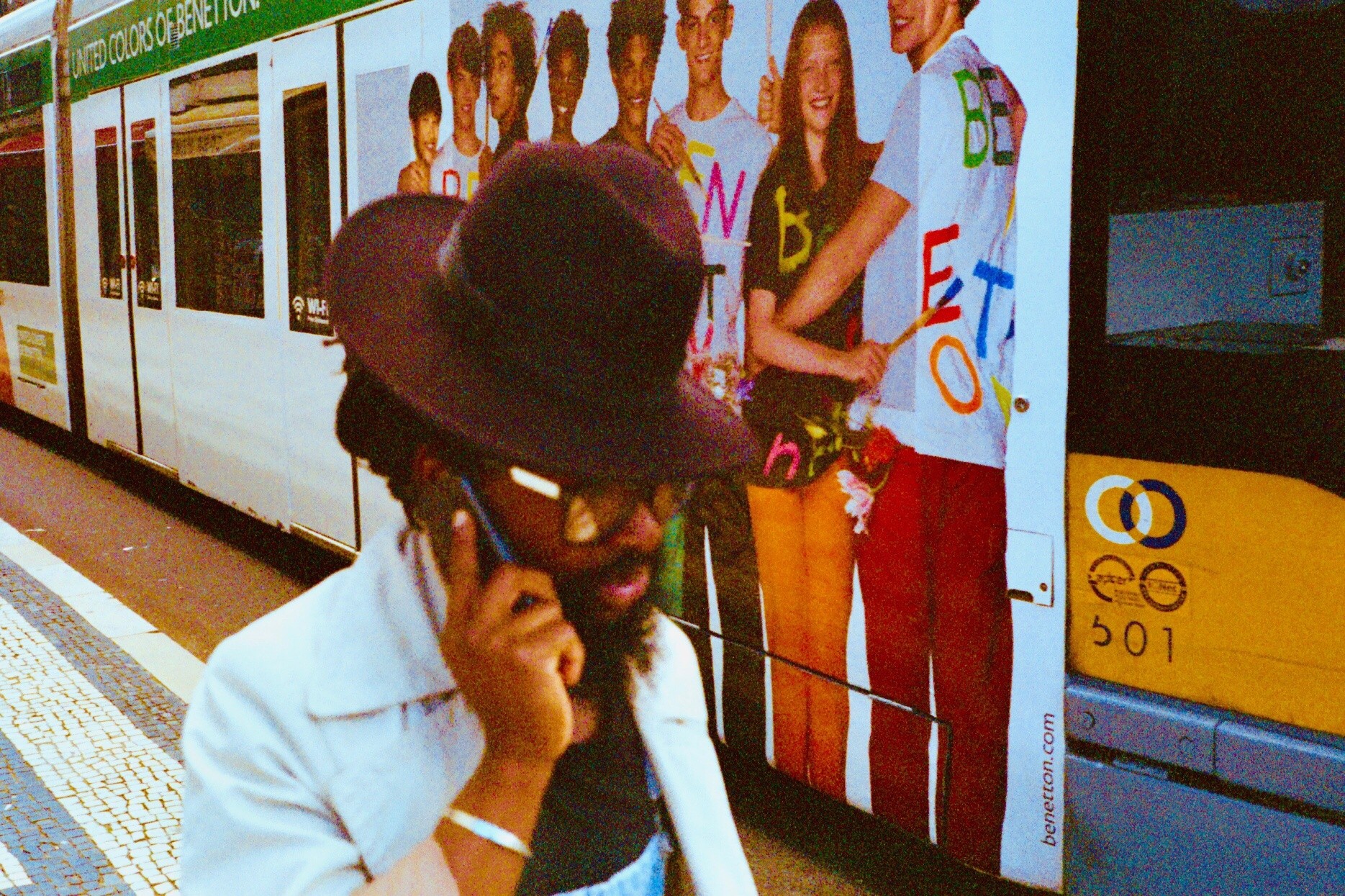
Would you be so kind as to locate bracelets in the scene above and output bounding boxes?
[430,800,541,865]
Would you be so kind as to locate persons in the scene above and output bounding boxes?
[593,0,689,169]
[391,1,589,218]
[658,0,1035,877]
[182,259,760,896]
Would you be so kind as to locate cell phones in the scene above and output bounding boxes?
[421,473,534,617]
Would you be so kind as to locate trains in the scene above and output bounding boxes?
[1,0,1345,894]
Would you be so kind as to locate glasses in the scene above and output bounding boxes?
[447,444,692,545]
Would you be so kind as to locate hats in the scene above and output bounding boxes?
[319,138,759,481]
